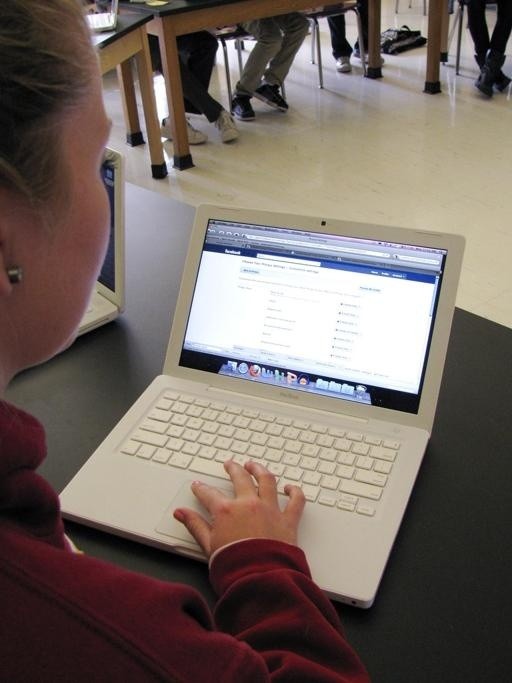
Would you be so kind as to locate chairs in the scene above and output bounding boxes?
[214,16,287,117]
[301,4,365,90]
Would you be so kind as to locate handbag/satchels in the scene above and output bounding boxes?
[380,25,428,55]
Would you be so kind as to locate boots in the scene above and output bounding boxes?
[474,52,512,92]
[474,48,507,99]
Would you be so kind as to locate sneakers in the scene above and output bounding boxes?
[160,117,208,145]
[353,47,385,65]
[230,92,256,120]
[252,83,289,111]
[335,56,352,72]
[214,109,240,143]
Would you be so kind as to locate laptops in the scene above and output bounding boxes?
[73,147,126,341]
[86,1,122,31]
[57,205,466,611]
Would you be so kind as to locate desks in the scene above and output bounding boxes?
[105,1,384,172]
[82,1,170,185]
[5,176,509,680]
[423,0,450,95]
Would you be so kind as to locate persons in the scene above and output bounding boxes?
[325,0,385,72]
[231,10,310,120]
[459,0,511,97]
[2,0,369,683]
[147,28,240,145]
